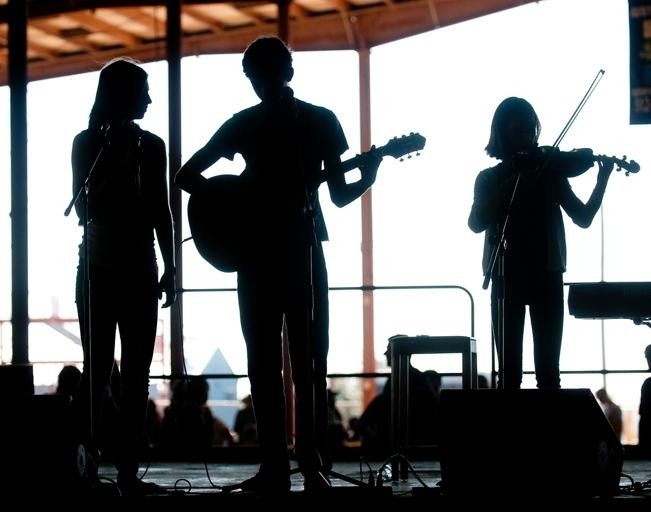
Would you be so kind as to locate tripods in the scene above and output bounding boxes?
[222,192,368,487]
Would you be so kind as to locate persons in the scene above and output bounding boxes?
[172,34,385,492]
[465,93,618,392]
[55,330,651,454]
[64,56,183,498]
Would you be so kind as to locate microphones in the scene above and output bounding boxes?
[513,150,530,160]
[93,113,120,134]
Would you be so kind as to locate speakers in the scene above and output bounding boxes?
[440,387,626,497]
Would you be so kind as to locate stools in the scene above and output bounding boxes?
[389,335,479,484]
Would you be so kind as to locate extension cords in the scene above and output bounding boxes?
[360,474,394,496]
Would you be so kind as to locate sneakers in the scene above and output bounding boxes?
[304,474,335,493]
[231,474,292,494]
[118,473,166,495]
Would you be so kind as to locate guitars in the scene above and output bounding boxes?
[188,132,426,273]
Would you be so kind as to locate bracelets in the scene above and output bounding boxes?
[166,266,178,273]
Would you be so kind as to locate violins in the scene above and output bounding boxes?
[528,146,642,177]
